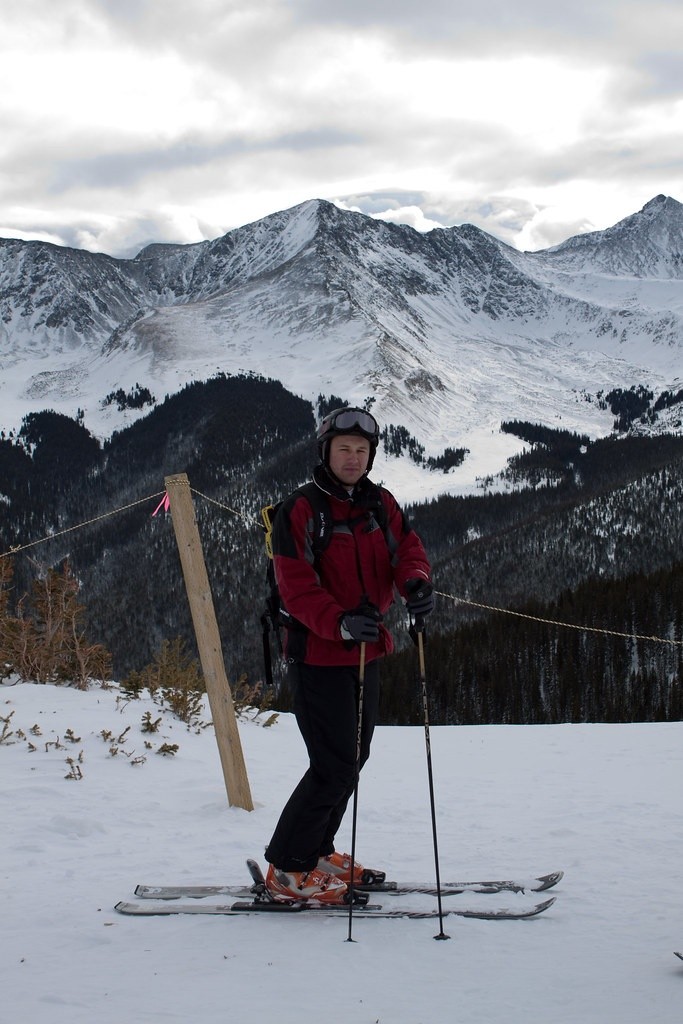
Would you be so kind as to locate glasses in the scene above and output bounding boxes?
[333,410,376,433]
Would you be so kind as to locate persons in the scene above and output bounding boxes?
[260,407,435,905]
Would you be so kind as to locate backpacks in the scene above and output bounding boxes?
[260,481,333,684]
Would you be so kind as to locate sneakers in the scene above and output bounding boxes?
[265,852,364,904]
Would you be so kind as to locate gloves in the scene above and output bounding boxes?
[405,579,436,617]
[340,607,384,643]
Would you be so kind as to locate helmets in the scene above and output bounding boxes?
[318,408,380,463]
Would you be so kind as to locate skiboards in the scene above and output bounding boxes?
[135,869,564,900]
[114,899,554,919]
